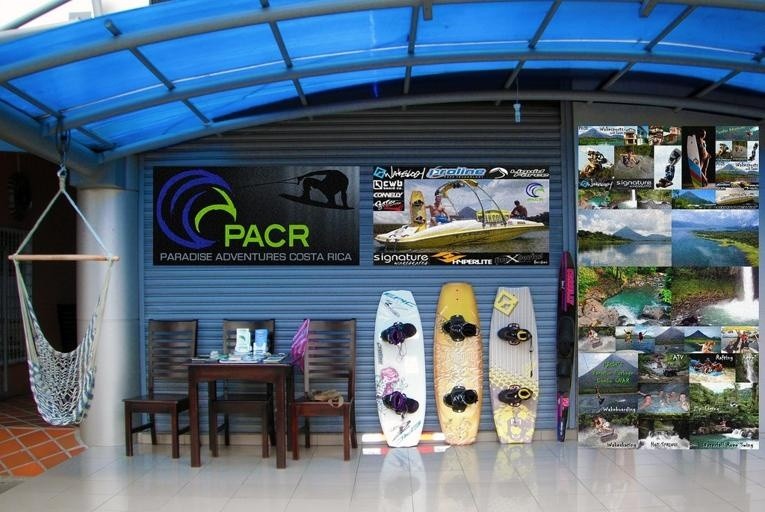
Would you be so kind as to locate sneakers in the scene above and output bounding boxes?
[498,388,533,403]
[442,321,477,337]
[413,216,426,224]
[498,327,533,342]
[381,323,416,346]
[443,390,478,406]
[412,200,425,208]
[382,392,419,413]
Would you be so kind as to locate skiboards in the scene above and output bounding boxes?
[555,252,577,442]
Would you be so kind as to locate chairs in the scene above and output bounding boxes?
[121,318,357,462]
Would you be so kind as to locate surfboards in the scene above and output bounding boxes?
[687,134,703,187]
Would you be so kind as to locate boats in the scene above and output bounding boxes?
[375,179,546,251]
[689,359,723,377]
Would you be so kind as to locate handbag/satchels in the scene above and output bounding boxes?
[306,390,344,408]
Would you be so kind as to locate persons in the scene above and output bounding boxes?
[509,198,529,220]
[584,124,761,189]
[425,194,452,227]
[587,325,755,443]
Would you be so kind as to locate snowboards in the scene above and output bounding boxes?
[433,282,483,446]
[409,190,427,234]
[488,286,539,444]
[373,290,426,447]
